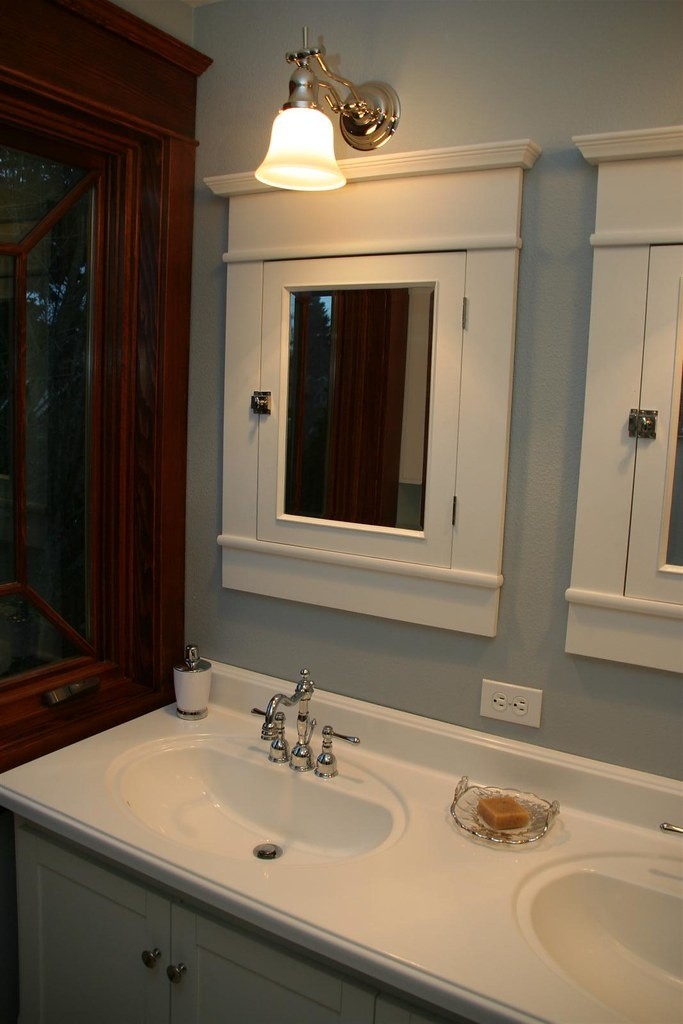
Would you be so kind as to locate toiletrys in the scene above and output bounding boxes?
[172,643,213,722]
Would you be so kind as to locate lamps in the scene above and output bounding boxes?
[254,24,400,192]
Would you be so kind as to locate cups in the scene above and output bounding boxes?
[173,644,212,720]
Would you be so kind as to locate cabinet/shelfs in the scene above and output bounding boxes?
[12,811,463,1024]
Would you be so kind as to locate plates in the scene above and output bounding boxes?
[451,776,560,845]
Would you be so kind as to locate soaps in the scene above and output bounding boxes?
[477,797,531,831]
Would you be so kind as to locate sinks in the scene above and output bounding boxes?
[120,745,395,866]
[529,870,683,1024]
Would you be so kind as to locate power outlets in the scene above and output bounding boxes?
[479,679,543,729]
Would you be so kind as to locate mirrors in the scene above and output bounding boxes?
[256,248,468,569]
[623,244,683,605]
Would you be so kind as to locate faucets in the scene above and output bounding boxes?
[265,668,317,772]
